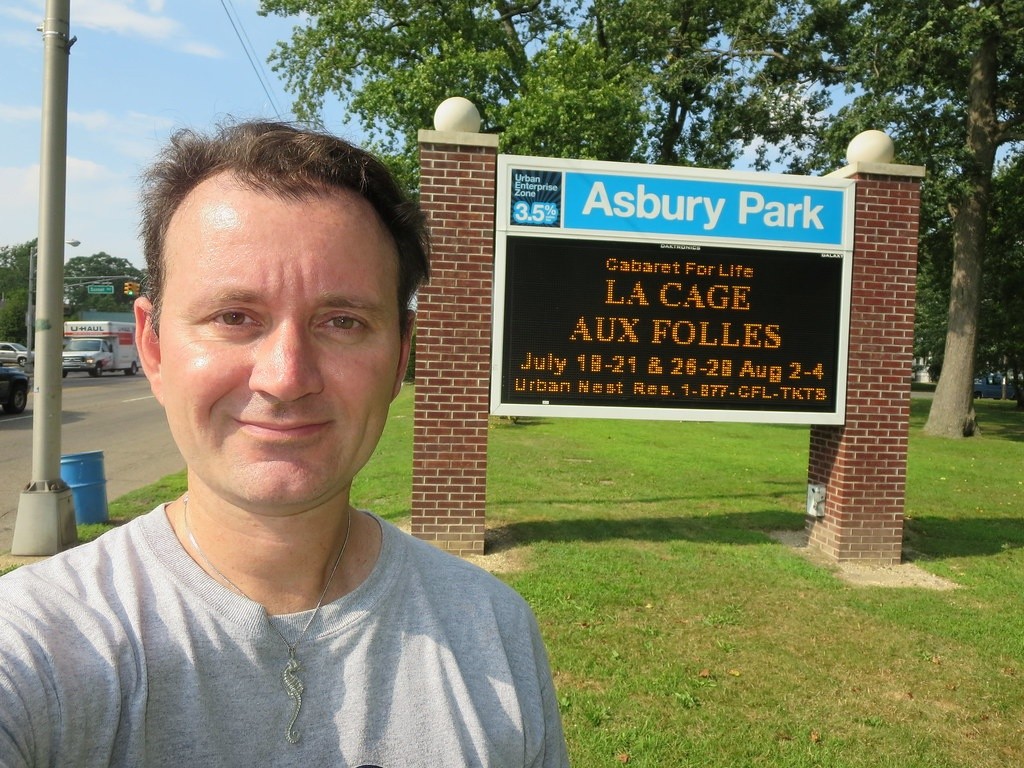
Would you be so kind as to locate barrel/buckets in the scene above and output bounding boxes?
[60,449,109,524]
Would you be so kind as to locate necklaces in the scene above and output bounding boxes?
[183,498,350,744]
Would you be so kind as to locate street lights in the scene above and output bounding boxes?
[26,242,80,373]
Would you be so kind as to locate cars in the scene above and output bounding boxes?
[0,363,31,415]
[0,343,34,367]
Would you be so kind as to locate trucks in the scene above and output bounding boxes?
[62,321,143,378]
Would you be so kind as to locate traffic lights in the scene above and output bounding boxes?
[134,283,140,296]
[128,283,133,295]
[124,283,128,294]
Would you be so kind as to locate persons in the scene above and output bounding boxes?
[0,122,569,768]
